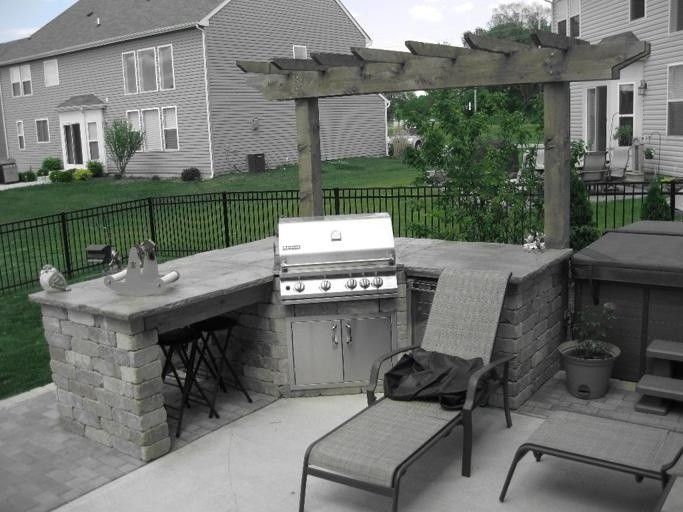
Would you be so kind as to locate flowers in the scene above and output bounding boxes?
[557,303,620,356]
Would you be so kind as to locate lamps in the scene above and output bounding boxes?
[638,80,647,95]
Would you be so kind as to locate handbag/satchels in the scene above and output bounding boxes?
[384,348,483,410]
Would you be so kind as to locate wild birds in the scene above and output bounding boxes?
[140,239,157,261]
[39,263,72,293]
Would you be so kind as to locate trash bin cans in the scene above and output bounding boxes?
[0,159,19,184]
[248,153,265,172]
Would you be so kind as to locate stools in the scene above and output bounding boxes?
[158,316,252,437]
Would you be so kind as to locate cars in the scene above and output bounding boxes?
[389,127,426,151]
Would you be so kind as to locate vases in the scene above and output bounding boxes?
[558,340,620,401]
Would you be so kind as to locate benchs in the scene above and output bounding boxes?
[632,339,682,416]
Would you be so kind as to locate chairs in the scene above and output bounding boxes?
[527,146,631,194]
[500,410,683,501]
[300,266,516,512]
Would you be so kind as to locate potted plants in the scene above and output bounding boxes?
[643,147,655,159]
[614,125,632,146]
